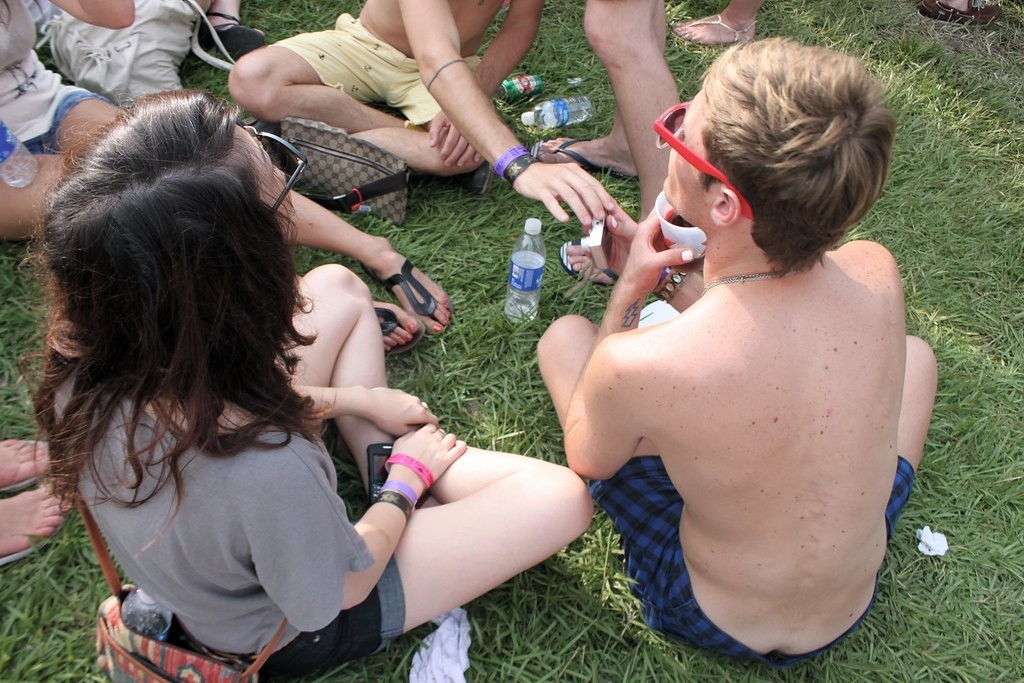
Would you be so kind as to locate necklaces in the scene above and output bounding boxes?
[709,262,801,286]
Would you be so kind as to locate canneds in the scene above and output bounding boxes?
[497,75,543,102]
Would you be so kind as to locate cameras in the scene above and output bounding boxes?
[581,210,613,269]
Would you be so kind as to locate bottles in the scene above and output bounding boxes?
[521,95,594,129]
[0,120,38,189]
[504,218,546,323]
[121,588,172,642]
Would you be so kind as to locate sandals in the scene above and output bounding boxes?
[198,8,265,57]
[670,13,757,48]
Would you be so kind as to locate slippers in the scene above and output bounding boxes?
[560,237,627,287]
[356,247,454,337]
[541,136,642,181]
[370,299,425,357]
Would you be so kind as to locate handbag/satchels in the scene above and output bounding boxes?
[91,583,259,683]
[279,115,411,225]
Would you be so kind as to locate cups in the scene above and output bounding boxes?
[654,189,707,259]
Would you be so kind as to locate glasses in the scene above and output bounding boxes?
[246,126,308,213]
[653,103,757,221]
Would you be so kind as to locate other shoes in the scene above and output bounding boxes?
[918,0,1003,27]
[465,163,494,197]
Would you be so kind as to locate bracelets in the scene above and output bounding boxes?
[372,490,413,522]
[654,265,672,288]
[381,478,418,511]
[501,153,538,183]
[493,144,531,178]
[387,452,437,486]
[654,270,691,304]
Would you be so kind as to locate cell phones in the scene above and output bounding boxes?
[368,444,394,505]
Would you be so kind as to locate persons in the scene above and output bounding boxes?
[0,0,1012,356]
[535,38,939,661]
[26,91,596,683]
[0,439,71,565]
